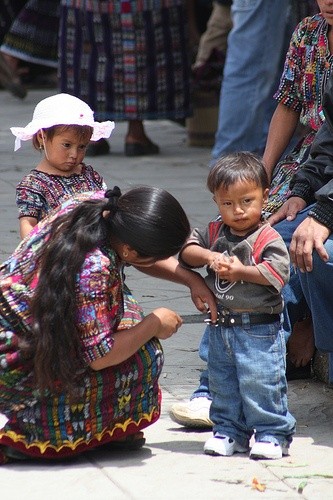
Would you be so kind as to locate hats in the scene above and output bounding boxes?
[10,93,116,151]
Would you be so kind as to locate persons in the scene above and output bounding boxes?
[256,0,333,222]
[178,152,297,460]
[0,0,321,181]
[0,185,218,463]
[10,93,108,240]
[266,53,333,387]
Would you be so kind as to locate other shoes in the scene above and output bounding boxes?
[94,431,146,450]
[0,53,27,98]
[0,444,32,459]
[286,355,311,379]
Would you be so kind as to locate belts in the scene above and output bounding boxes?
[204,308,280,328]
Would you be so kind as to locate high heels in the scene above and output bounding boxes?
[125,121,160,156]
[86,138,109,155]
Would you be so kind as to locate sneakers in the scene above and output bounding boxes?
[249,440,288,459]
[169,396,216,428]
[204,432,248,456]
